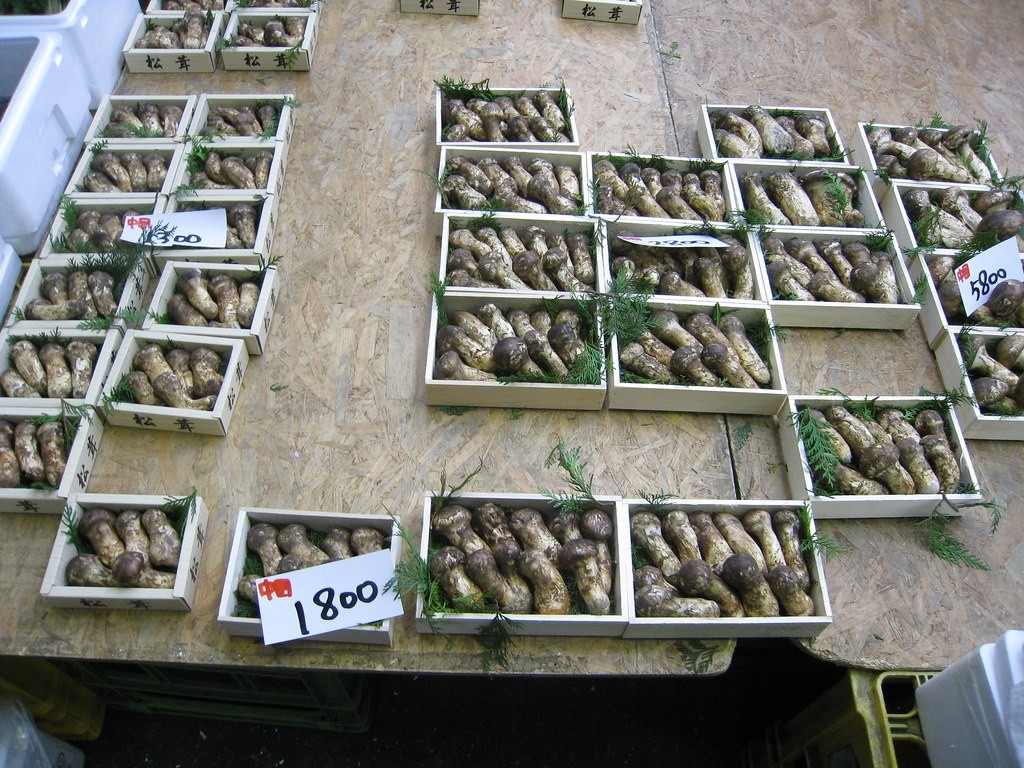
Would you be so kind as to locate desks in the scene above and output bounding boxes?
[0,0,1023,673]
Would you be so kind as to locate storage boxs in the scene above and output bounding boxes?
[0,0,399,736]
[418,86,1024,768]
[401,0,481,18]
[562,0,642,25]
